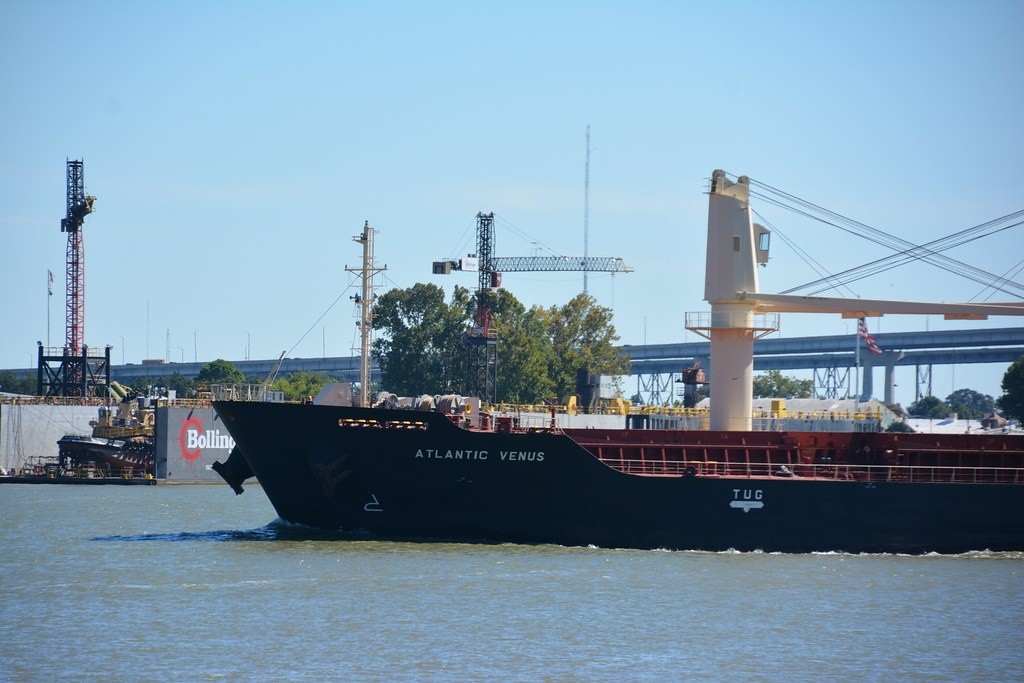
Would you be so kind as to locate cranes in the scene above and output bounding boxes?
[255,351,287,400]
[59,157,95,394]
[431,211,639,404]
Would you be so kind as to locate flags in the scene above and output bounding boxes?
[859,317,882,355]
[48,273,54,295]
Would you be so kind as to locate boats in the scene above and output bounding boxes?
[57,383,176,472]
[206,170,1024,554]
